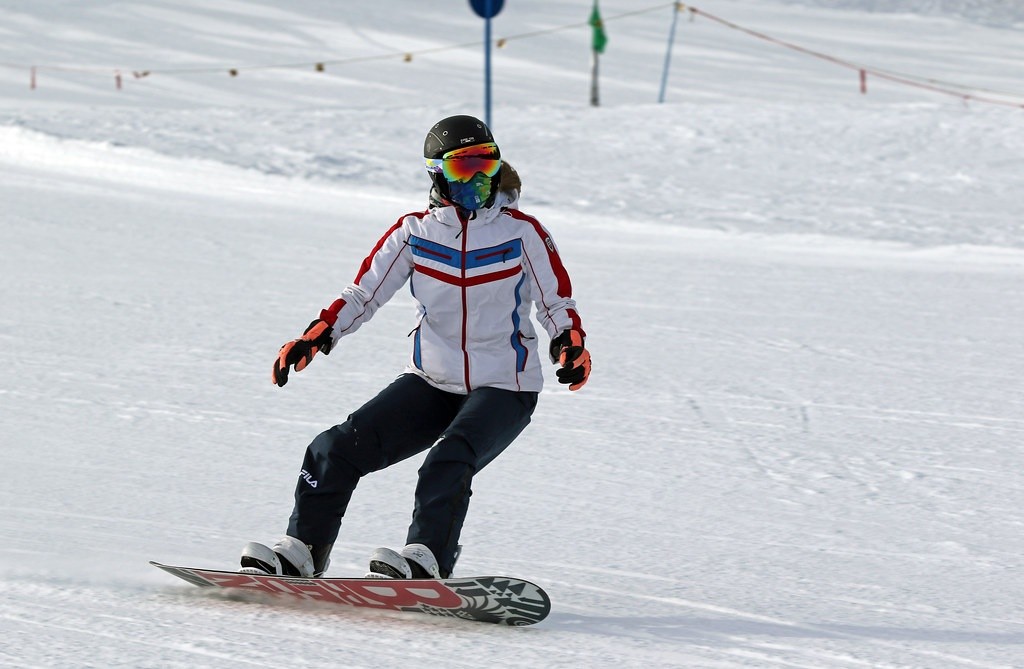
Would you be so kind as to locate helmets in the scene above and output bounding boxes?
[424,115,502,206]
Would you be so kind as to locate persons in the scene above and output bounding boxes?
[240,115,592,578]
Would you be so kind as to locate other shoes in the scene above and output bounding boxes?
[369,543,441,581]
[241,537,317,582]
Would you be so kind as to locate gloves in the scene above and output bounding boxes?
[272,320,331,386]
[555,329,591,391]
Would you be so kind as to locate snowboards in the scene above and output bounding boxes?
[148,558,554,628]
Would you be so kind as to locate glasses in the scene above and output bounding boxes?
[441,142,502,184]
